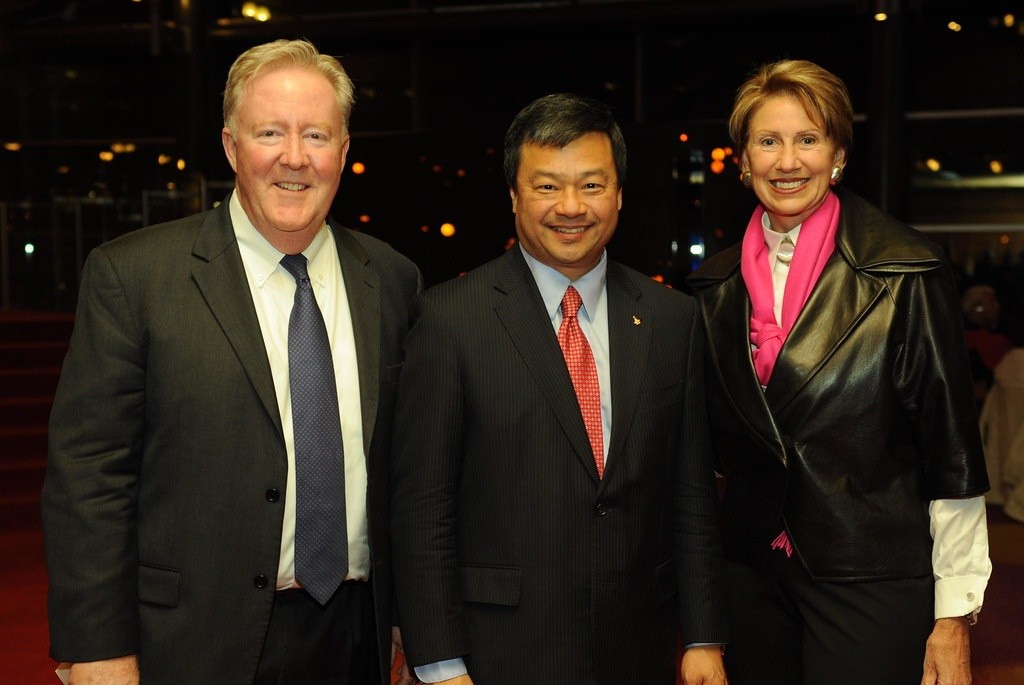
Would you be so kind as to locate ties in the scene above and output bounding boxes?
[277,248,354,606]
[555,283,604,480]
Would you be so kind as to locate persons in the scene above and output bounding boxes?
[41,38,425,684]
[384,90,726,684]
[697,56,992,681]
